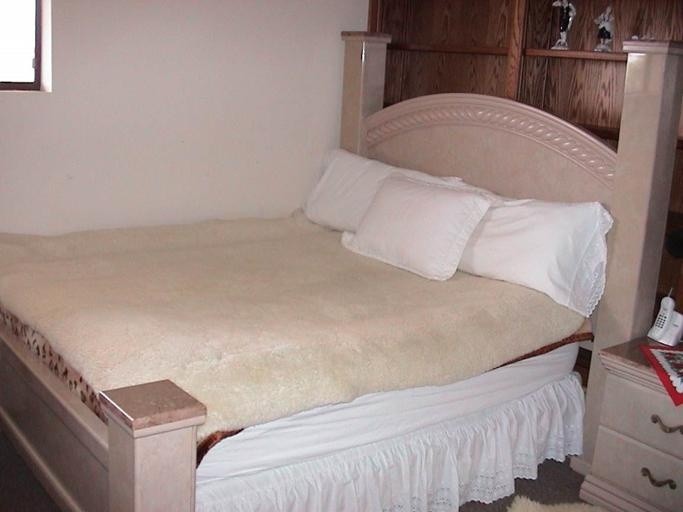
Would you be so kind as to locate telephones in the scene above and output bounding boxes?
[647,288,683,347]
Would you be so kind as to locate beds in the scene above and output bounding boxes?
[0,23,683,511]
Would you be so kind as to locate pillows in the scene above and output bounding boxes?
[299,146,614,316]
[337,172,490,282]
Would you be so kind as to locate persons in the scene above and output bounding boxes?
[591,1,615,52]
[550,0,576,48]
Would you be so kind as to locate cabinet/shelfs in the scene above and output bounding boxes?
[360,0,682,316]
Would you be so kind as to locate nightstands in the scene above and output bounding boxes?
[577,328,683,511]
